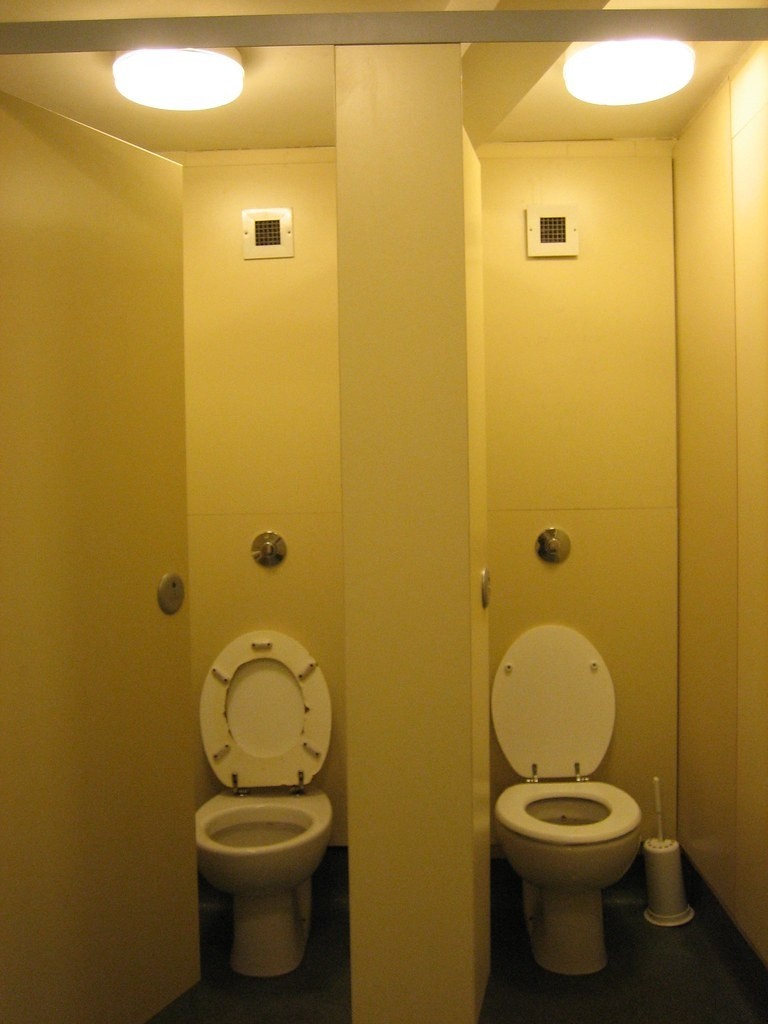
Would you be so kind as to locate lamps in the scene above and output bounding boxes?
[112,44,245,109]
[562,37,696,104]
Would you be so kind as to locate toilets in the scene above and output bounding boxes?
[193,625,335,980]
[492,624,643,976]
